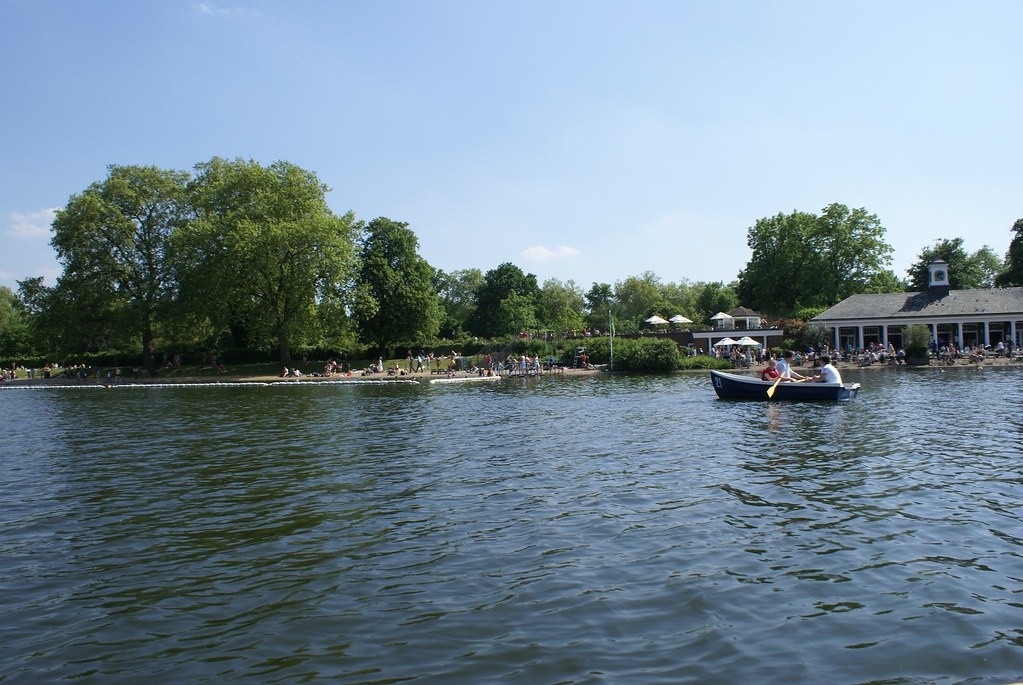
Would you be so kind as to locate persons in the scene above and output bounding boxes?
[806,356,842,384]
[408,349,495,379]
[158,352,225,372]
[709,346,771,370]
[0,361,35,383]
[44,362,137,384]
[281,357,407,378]
[776,350,805,382]
[928,336,1023,365]
[506,353,539,374]
[761,360,780,382]
[846,341,906,366]
[517,327,601,339]
[793,341,846,365]
[686,345,703,358]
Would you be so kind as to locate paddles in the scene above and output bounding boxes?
[796,374,820,383]
[766,371,786,398]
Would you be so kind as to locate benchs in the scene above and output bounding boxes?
[387,371,407,375]
[431,369,447,373]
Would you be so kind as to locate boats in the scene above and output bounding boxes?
[708,370,861,400]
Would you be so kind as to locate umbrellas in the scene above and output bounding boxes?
[710,312,733,328]
[736,336,760,346]
[645,316,669,333]
[669,315,693,326]
[714,337,737,350]
